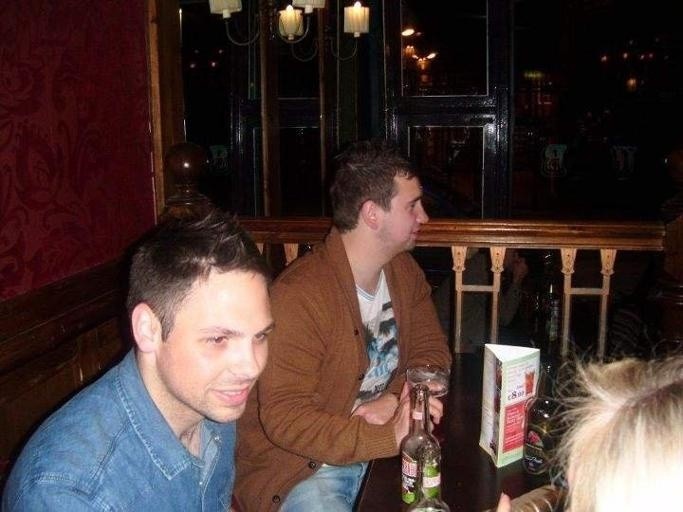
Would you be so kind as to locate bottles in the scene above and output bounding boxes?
[406,442,451,511]
[527,282,563,351]
[399,385,444,509]
[520,361,560,474]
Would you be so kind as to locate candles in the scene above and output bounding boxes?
[342,1,368,39]
[277,5,305,41]
[291,0,326,15]
[208,1,242,20]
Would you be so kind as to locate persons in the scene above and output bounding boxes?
[232,135,457,512]
[0,184,275,510]
[491,339,683,511]
